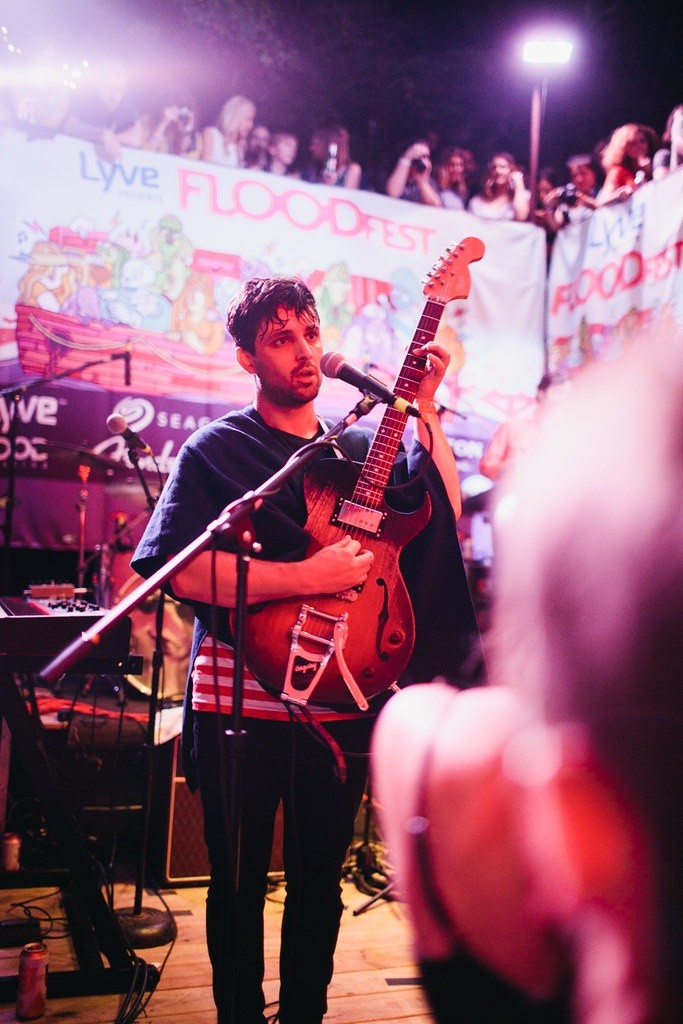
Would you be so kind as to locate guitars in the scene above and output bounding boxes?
[224,233,490,708]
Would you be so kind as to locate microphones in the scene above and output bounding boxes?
[317,352,422,417]
[124,337,133,384]
[105,412,157,455]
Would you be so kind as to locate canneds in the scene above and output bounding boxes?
[15,943,49,1018]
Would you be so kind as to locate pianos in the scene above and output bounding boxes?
[0,589,142,969]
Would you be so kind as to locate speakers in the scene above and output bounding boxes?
[147,734,282,885]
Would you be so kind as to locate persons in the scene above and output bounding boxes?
[129,274,464,1024]
[368,328,683,1024]
[0,94,682,268]
